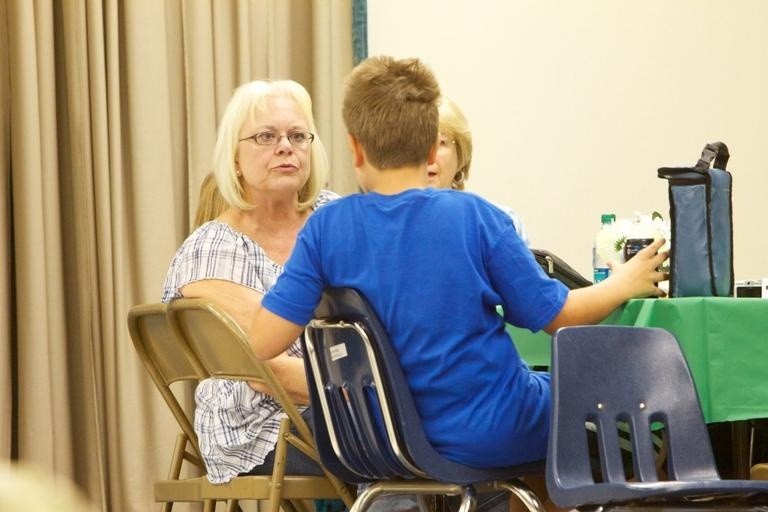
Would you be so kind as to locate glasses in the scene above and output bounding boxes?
[239,132,314,145]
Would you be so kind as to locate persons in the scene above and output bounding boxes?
[424,94,527,256]
[249,56,676,510]
[173,80,430,512]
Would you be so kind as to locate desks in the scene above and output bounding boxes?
[504,296,766,479]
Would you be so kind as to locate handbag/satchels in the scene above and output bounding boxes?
[531,248,593,289]
[669,142,733,298]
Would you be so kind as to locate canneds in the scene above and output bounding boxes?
[626,239,658,298]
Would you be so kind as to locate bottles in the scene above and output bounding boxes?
[590,213,669,298]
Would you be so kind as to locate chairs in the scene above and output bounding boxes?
[298,286,542,510]
[542,325,767,511]
[165,297,353,512]
[127,300,296,512]
